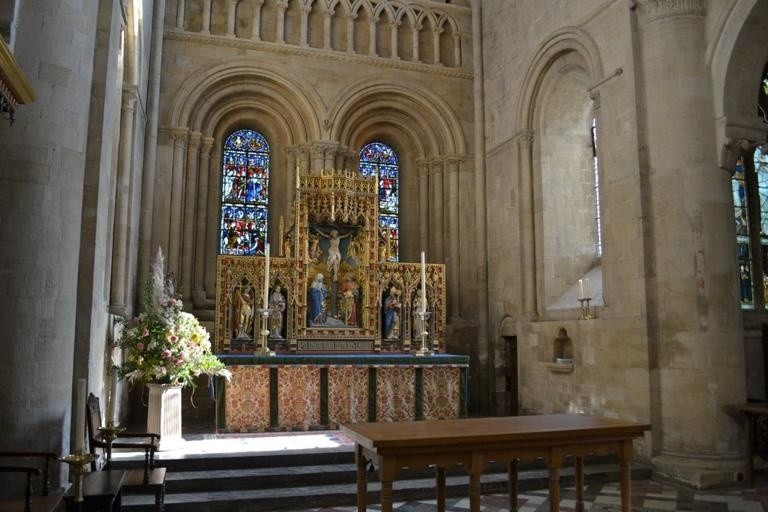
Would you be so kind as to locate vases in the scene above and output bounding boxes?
[144,384,186,451]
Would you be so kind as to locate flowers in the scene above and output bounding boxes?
[108,272,233,388]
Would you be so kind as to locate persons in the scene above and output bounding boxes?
[310,273,328,325]
[284,233,295,259]
[269,284,286,339]
[313,226,353,279]
[384,285,401,338]
[234,285,254,339]
[412,287,428,338]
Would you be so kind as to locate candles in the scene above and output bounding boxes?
[585,279,589,298]
[578,279,583,298]
[421,251,426,312]
[263,243,270,309]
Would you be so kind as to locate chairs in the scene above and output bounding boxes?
[0,451,63,512]
[86,392,166,512]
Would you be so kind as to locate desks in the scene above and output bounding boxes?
[53,470,127,512]
[339,413,652,512]
[737,400,768,487]
